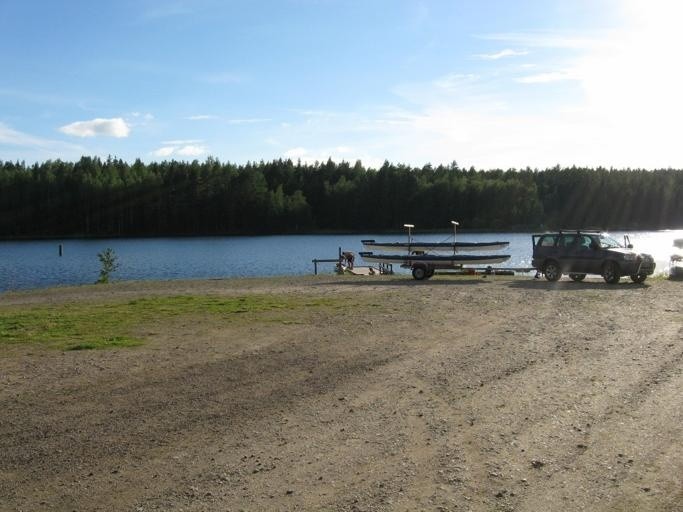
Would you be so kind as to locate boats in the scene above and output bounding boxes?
[358,238,513,265]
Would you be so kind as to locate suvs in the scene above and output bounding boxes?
[530,228,656,284]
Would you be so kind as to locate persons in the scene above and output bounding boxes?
[343,251,354,269]
